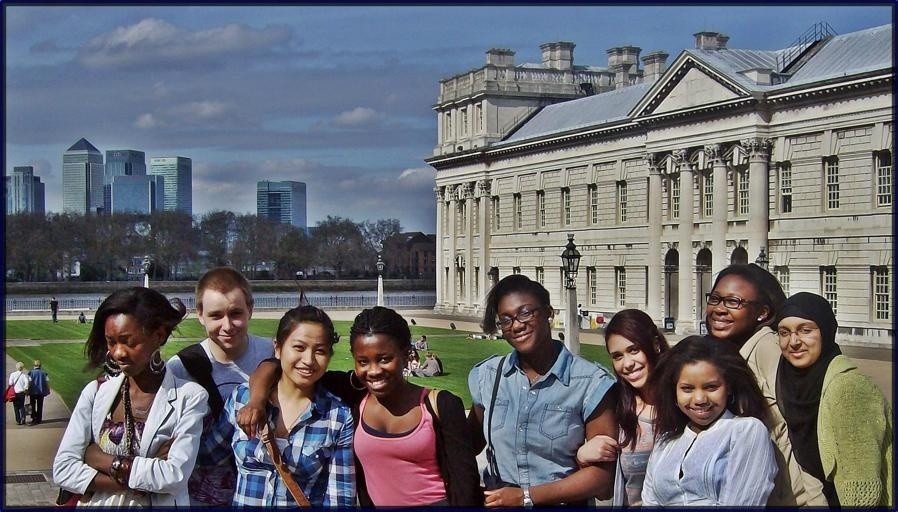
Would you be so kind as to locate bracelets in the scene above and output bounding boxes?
[108,455,130,487]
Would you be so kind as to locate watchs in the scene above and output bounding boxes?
[523,486,534,508]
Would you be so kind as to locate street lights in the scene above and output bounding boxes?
[374,255,385,307]
[140,254,152,288]
[558,232,584,359]
[755,245,768,272]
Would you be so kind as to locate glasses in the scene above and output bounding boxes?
[496,308,539,329]
[705,293,753,309]
[780,327,820,335]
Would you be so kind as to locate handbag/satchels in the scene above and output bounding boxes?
[6,385,15,402]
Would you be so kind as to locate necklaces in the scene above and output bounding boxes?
[528,353,553,389]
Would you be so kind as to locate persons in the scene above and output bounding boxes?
[403,329,504,379]
[704,263,841,509]
[8,361,32,425]
[167,268,275,508]
[575,309,670,509]
[775,291,894,509]
[49,297,88,325]
[235,306,484,509]
[196,305,357,509]
[52,285,209,508]
[464,275,620,509]
[24,360,50,426]
[640,335,779,508]
